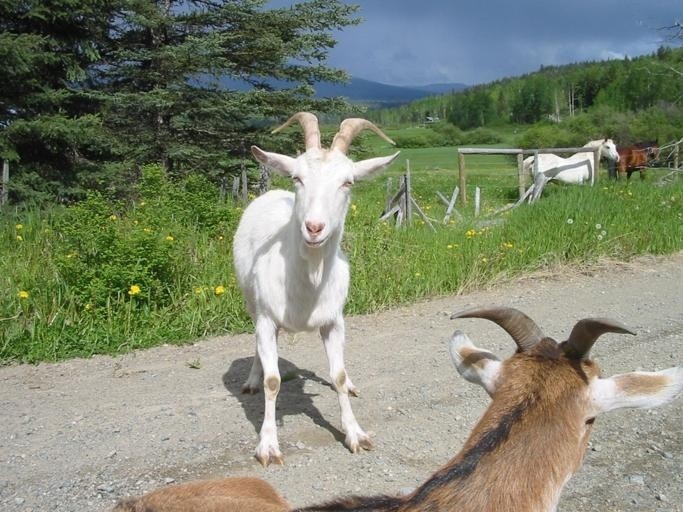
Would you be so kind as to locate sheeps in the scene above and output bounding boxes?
[110,304,683,512]
[232,110,403,469]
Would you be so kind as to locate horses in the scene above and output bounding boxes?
[523,135,620,205]
[616,137,660,185]
